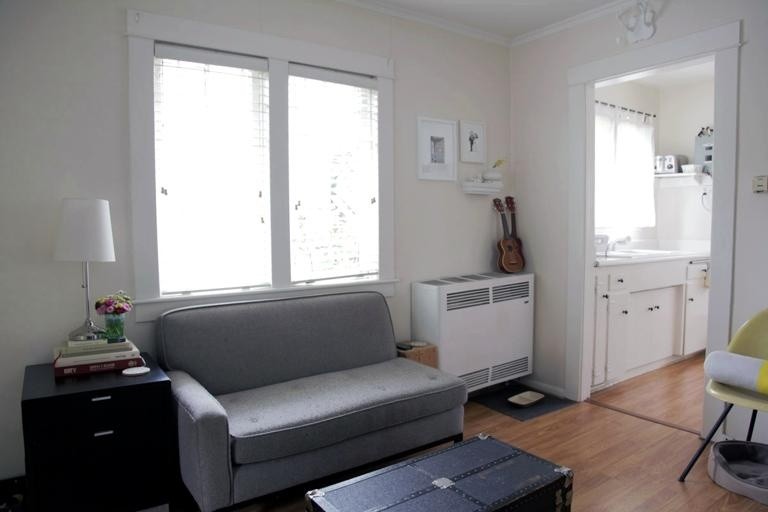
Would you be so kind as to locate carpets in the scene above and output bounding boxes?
[469,379,577,422]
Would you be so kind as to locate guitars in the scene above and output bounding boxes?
[506,196,525,270]
[493,198,523,273]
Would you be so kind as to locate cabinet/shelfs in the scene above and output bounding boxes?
[607,263,683,378]
[19,351,177,512]
[685,263,713,358]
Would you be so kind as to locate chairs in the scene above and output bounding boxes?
[678,305,767,482]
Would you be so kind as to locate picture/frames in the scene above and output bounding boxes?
[414,114,487,181]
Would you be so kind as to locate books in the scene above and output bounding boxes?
[52,338,147,381]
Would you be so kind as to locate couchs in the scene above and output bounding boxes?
[159,288,469,512]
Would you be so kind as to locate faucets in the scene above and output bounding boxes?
[604,240,626,257]
[612,236,631,249]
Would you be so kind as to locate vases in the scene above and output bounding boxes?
[104,315,124,339]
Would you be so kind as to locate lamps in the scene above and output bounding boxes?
[53,198,116,341]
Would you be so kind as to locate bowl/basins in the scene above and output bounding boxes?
[680,163,704,174]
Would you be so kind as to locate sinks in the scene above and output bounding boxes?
[605,255,630,261]
[621,250,642,255]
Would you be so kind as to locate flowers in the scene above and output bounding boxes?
[94,290,133,338]
